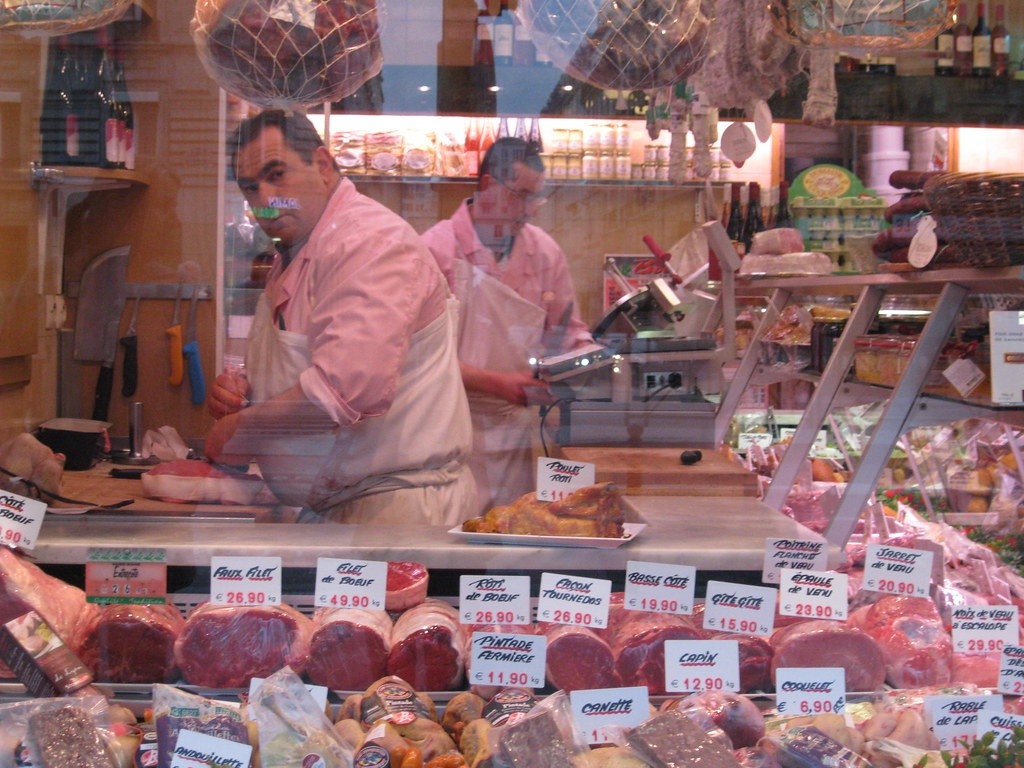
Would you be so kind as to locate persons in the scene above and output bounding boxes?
[419,136,595,519]
[204,108,486,597]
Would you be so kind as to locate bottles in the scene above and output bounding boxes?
[40,21,139,173]
[727,181,793,273]
[935,0,1010,76]
[468,114,541,180]
[475,1,535,69]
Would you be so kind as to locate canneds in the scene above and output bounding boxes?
[539,124,734,183]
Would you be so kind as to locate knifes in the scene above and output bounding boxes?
[164,284,185,385]
[120,289,142,396]
[183,286,206,406]
[72,245,131,420]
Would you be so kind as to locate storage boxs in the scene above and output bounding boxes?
[38,417,114,457]
[0,610,94,698]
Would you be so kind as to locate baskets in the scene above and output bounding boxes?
[923,172,1024,269]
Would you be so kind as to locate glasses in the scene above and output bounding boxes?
[493,175,547,206]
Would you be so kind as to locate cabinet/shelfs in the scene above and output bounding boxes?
[0,0,159,426]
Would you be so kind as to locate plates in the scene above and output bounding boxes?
[446,517,647,550]
